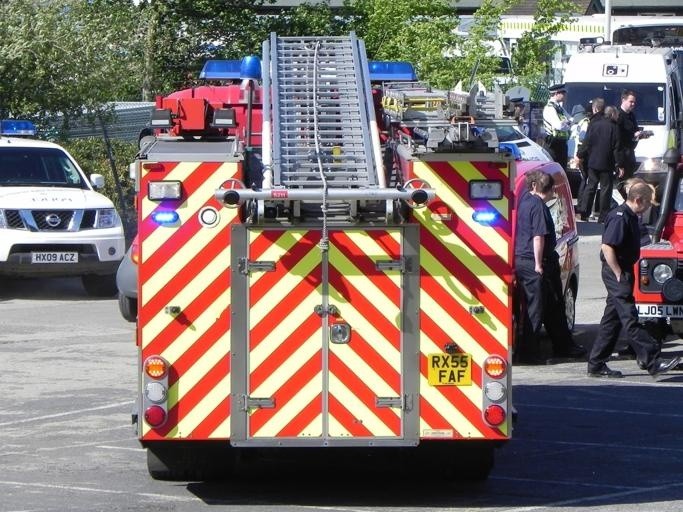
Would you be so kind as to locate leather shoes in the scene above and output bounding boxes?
[647,357,678,379]
[588,367,621,379]
[553,340,587,358]
[577,213,610,224]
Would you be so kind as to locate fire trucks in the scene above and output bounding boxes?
[125,32,515,464]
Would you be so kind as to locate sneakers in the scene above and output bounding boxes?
[519,351,554,364]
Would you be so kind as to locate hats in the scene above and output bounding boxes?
[548,85,567,96]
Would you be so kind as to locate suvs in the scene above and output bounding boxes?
[1,117,124,294]
[493,123,580,357]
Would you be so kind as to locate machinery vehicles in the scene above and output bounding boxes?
[615,45,683,365]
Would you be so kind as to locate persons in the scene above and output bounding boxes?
[511,83,681,379]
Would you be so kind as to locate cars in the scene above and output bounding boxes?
[111,226,139,323]
